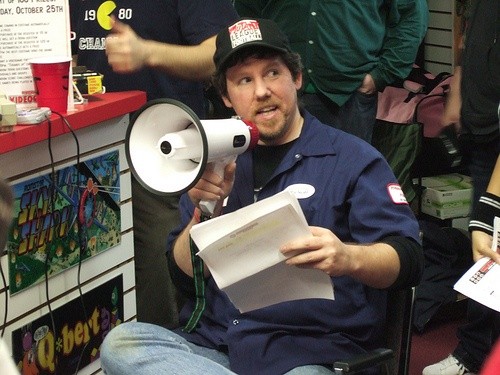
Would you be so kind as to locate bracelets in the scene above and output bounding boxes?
[468,190,500,237]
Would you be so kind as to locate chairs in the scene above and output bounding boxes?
[333,231,424,375]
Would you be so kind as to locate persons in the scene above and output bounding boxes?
[100,18,423,375]
[205,0,427,146]
[468,154,500,265]
[70,0,239,330]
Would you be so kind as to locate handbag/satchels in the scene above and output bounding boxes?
[377,60,454,218]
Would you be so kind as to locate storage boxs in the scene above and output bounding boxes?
[452,218,470,231]
[412,173,472,220]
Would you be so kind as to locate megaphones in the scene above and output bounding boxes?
[125,98,260,218]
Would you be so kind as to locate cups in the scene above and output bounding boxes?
[28,57,73,114]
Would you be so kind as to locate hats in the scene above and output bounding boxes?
[212,18,292,77]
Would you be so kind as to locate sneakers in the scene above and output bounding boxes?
[422,353,470,375]
